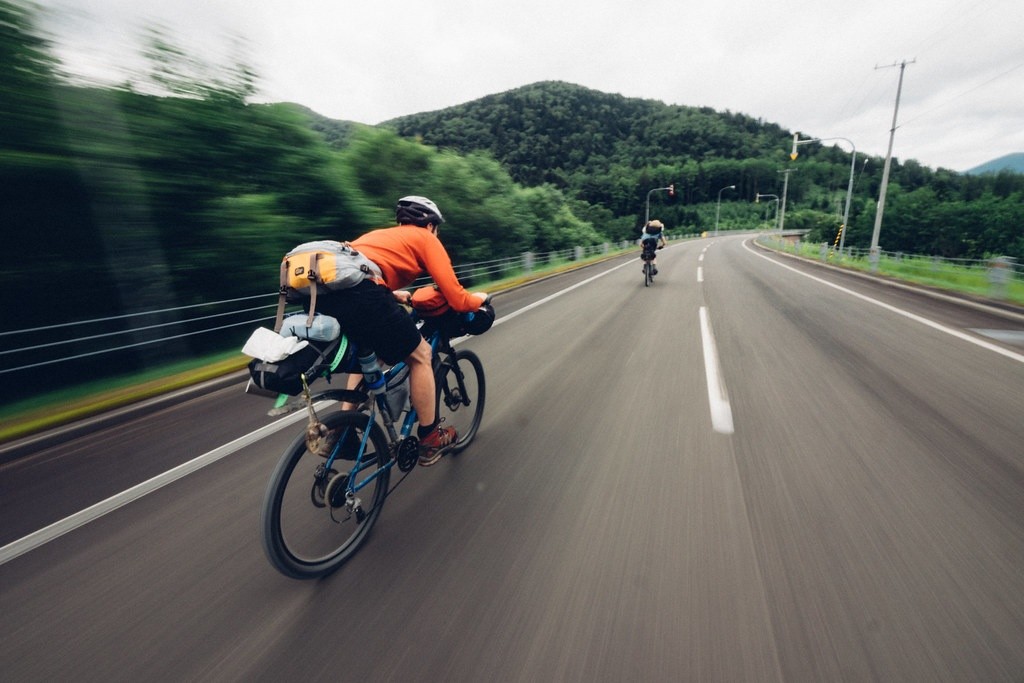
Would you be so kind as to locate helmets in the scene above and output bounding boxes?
[395,196,445,225]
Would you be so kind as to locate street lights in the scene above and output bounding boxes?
[789,133,855,255]
[645,185,674,225]
[755,193,779,230]
[715,185,735,237]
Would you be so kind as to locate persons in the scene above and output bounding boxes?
[303,196,488,467]
[640,220,666,275]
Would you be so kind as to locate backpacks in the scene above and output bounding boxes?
[646,219,661,234]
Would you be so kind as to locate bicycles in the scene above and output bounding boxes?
[641,244,665,286]
[260,291,491,582]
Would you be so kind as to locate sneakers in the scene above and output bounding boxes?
[319,428,367,460]
[418,417,458,466]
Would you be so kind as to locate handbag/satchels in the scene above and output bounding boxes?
[279,239,366,296]
